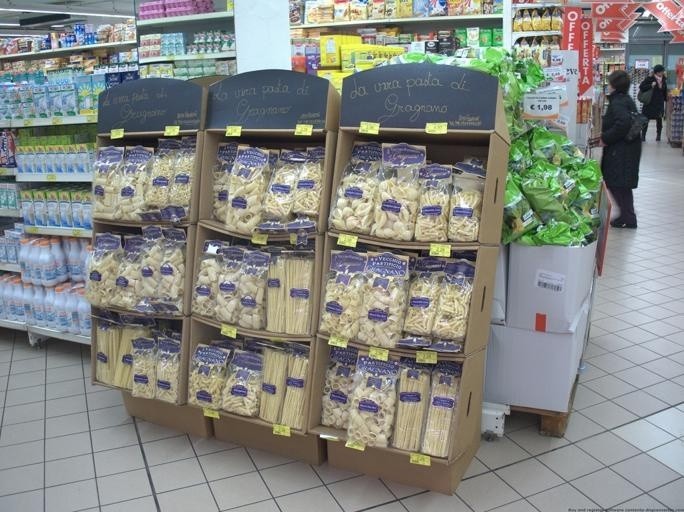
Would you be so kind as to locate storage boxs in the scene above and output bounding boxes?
[483,236,601,417]
[90,75,215,442]
[183,69,341,466]
[308,64,513,496]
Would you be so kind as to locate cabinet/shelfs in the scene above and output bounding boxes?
[1,0,236,349]
[290,0,630,162]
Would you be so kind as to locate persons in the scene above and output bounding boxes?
[638,64,669,142]
[598,69,641,228]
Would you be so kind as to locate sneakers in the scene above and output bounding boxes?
[610,216,637,228]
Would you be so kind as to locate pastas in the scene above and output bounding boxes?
[88,136,485,457]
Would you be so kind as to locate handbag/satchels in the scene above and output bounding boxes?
[638,86,655,104]
[623,111,649,144]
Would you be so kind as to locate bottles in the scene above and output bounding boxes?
[17,236,92,285]
[0,270,90,337]
